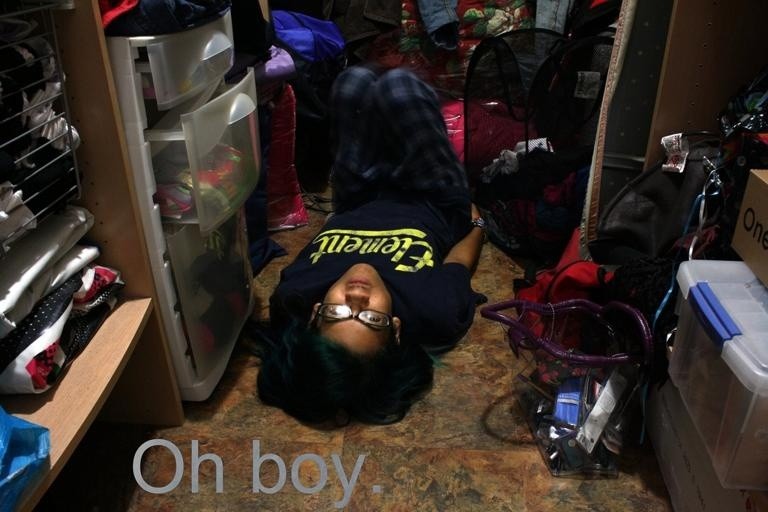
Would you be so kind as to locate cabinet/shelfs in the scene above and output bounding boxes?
[0,0,183,512]
[58,0,261,425]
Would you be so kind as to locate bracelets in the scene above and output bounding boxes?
[471,215,491,245]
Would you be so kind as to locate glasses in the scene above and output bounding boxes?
[310,304,396,330]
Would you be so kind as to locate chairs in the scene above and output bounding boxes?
[465,28,596,321]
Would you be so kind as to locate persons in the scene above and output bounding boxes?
[256,65,490,430]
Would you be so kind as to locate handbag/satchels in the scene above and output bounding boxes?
[481,298,654,479]
[272,11,347,194]
[516,228,622,352]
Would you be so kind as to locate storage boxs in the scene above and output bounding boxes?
[655,380,768,512]
[731,171,768,291]
[670,259,768,490]
